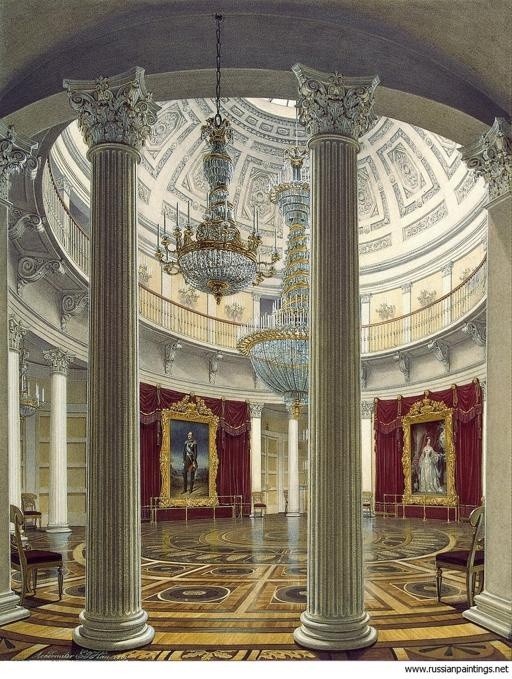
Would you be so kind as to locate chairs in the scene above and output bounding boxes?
[10,504,65,606]
[434,504,485,608]
[361,491,373,517]
[251,491,267,519]
[21,492,42,533]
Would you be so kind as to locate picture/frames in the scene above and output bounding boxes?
[401,397,459,507]
[159,394,220,508]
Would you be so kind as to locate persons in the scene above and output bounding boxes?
[181,432,198,494]
[418,435,446,493]
